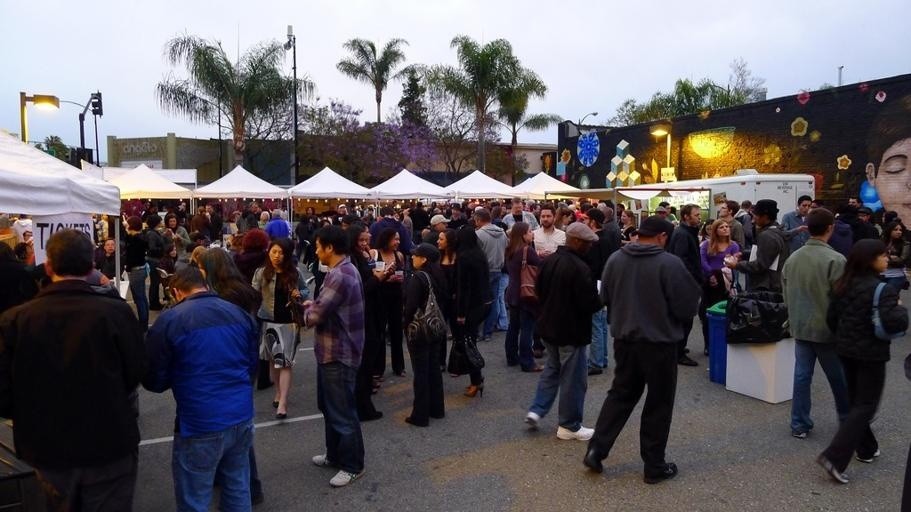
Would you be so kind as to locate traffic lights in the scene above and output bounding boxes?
[90,94,102,114]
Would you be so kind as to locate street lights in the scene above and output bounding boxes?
[651,126,678,178]
[282,24,301,182]
[16,94,61,144]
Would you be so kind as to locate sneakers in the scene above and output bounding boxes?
[534,349,542,358]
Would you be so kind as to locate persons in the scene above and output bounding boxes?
[402,196,911,431]
[193,248,266,505]
[0,227,150,509]
[1,194,454,428]
[813,239,911,483]
[863,95,910,235]
[301,226,367,488]
[522,222,604,442]
[584,216,705,486]
[143,266,263,512]
[779,209,852,440]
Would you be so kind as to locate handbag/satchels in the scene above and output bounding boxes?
[872,311,905,340]
[404,295,447,344]
[902,278,910,290]
[519,265,540,299]
[290,301,304,325]
[127,266,147,285]
[448,336,485,375]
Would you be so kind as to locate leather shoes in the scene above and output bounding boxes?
[679,356,697,366]
[275,409,288,419]
[272,399,279,409]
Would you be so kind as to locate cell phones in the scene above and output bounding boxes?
[385,261,394,273]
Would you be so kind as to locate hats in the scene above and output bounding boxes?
[857,207,871,214]
[410,242,439,262]
[449,203,464,213]
[146,215,162,229]
[653,206,668,213]
[565,222,599,242]
[337,204,347,209]
[587,208,604,228]
[750,199,779,216]
[430,215,451,226]
[630,217,674,238]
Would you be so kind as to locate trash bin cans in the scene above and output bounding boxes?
[706,301,727,386]
[726,291,796,404]
